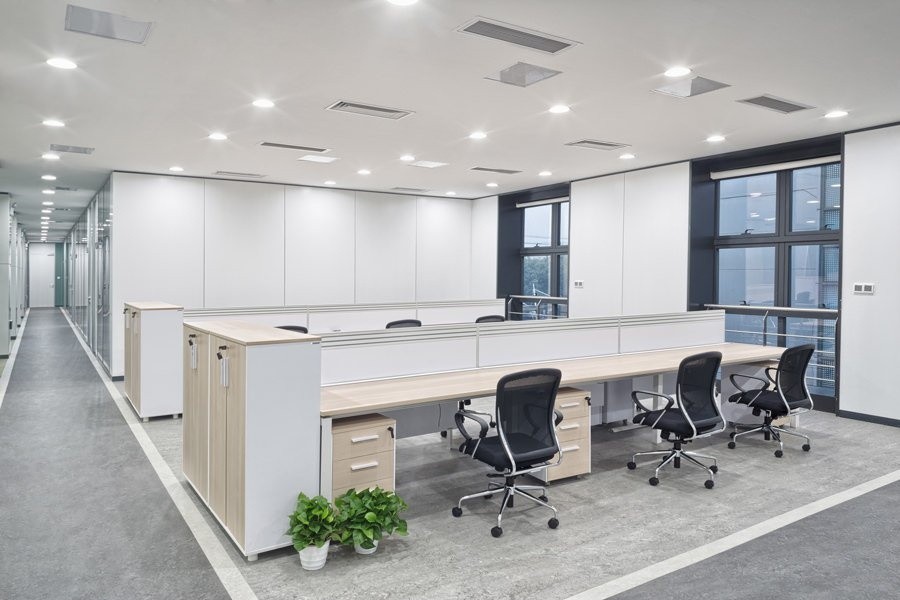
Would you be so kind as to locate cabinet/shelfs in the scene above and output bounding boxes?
[528,387,591,486]
[331,413,397,517]
[123,300,185,423]
[721,360,791,432]
[183,317,322,561]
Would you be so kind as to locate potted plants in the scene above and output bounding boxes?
[283,492,342,571]
[334,484,409,554]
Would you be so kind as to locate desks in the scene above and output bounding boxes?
[320,342,800,521]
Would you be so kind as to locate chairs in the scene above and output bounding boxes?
[626,351,728,490]
[451,368,564,538]
[274,314,505,334]
[730,343,816,459]
